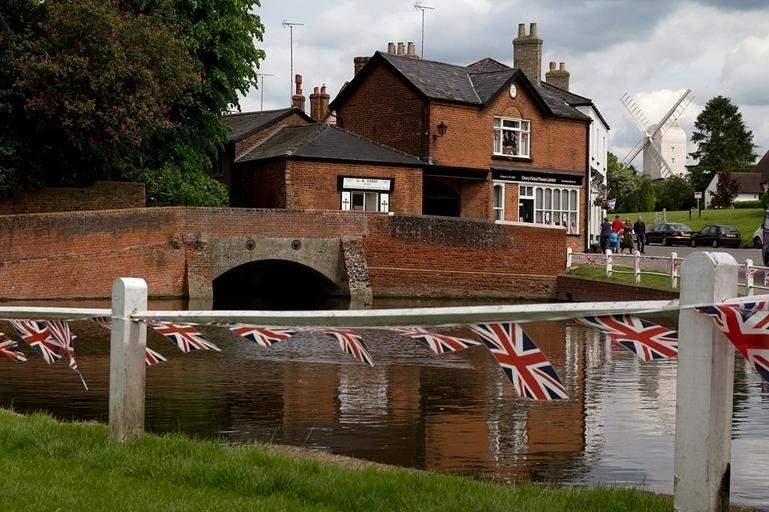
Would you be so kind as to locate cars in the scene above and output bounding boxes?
[605,222,637,242]
[643,222,693,246]
[750,225,764,248]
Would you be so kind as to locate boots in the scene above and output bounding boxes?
[621,248,632,254]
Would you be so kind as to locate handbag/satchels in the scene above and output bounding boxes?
[601,229,610,238]
[620,235,624,239]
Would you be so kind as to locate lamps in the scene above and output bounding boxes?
[433,121,447,142]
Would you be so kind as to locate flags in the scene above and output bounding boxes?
[465,322,569,402]
[572,315,678,363]
[316,328,374,367]
[208,323,297,349]
[134,320,221,354]
[693,300,769,383]
[608,198,616,210]
[764,271,769,284]
[744,268,759,276]
[390,328,482,356]
[90,317,167,367]
[0,319,88,390]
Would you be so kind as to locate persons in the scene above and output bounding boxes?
[599,215,646,254]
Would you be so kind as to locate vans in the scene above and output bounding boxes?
[687,224,742,248]
